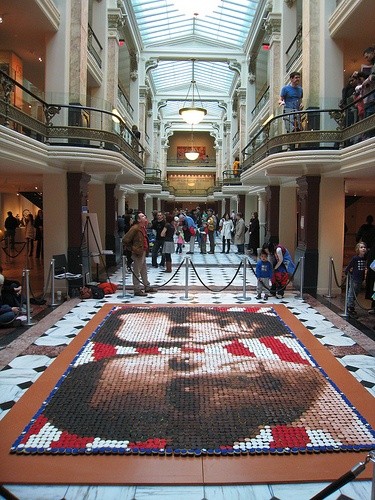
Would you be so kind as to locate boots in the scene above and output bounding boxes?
[208,246,214,254]
[166,262,171,273]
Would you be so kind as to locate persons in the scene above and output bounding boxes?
[256,252,273,300]
[355,215,375,250]
[233,157,240,178]
[33,210,43,258]
[42,353,375,447]
[132,126,140,141]
[345,242,375,319]
[219,213,234,254]
[247,212,260,256]
[118,206,246,273]
[24,214,37,257]
[3,211,27,250]
[278,72,304,151]
[0,266,33,328]
[91,308,292,348]
[334,47,375,148]
[122,212,158,296]
[160,215,175,273]
[268,237,296,299]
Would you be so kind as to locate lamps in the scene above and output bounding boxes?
[184,131,199,161]
[179,58,208,126]
[168,0,223,19]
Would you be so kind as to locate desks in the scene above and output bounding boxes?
[14,227,26,243]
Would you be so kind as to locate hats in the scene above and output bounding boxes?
[174,217,179,221]
[237,213,242,216]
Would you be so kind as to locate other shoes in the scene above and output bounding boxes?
[135,290,147,296]
[145,287,158,292]
[267,292,276,296]
[347,306,359,319]
[368,309,375,314]
[198,228,201,234]
[186,251,193,254]
[263,296,268,300]
[256,293,261,299]
[16,316,32,321]
[276,293,284,299]
[20,304,33,314]
[235,252,242,254]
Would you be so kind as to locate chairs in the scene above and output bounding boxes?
[53,254,83,302]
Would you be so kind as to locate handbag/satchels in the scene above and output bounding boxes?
[98,282,117,294]
[93,287,105,299]
[244,226,248,233]
[190,227,196,235]
[79,287,92,298]
